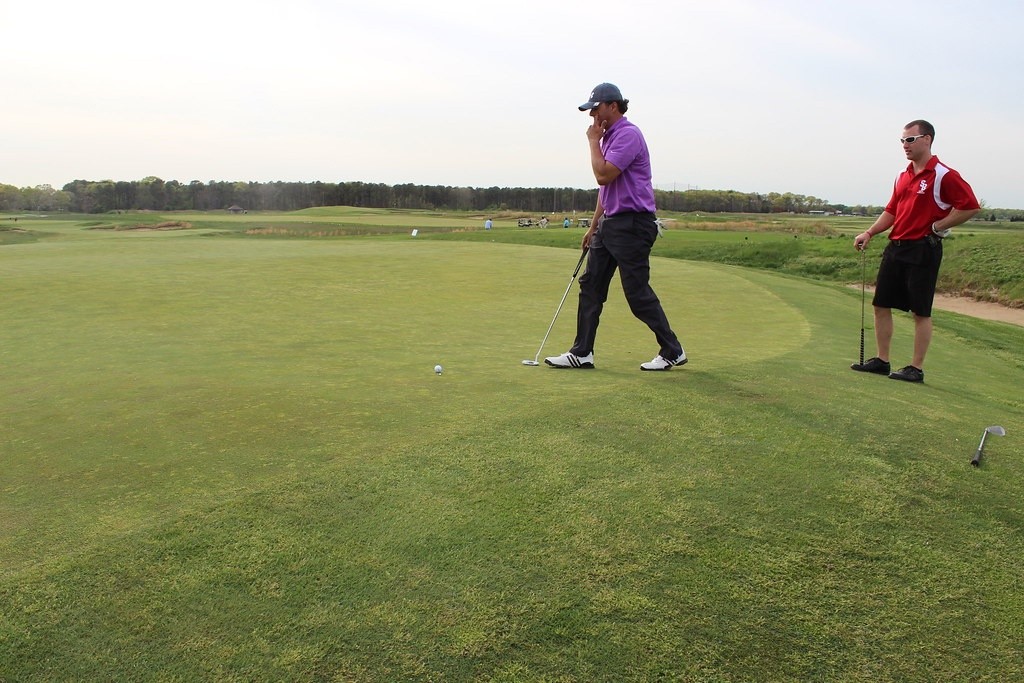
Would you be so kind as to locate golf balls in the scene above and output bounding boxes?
[434,365,442,373]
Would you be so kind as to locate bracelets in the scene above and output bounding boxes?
[865,231,872,238]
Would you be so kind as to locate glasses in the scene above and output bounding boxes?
[901,133,928,144]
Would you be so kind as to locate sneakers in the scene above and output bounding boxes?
[640,347,687,371]
[544,350,595,369]
[850,355,890,375]
[888,365,924,382]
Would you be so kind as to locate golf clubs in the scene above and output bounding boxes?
[522,243,590,366]
[971,426,1006,467]
[858,243,866,366]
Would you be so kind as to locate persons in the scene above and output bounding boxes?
[485,219,492,230]
[850,120,981,384]
[538,215,547,229]
[563,218,570,228]
[545,82,688,371]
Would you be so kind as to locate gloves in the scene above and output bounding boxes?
[654,218,668,238]
[932,222,952,238]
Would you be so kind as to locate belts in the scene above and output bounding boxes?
[892,239,927,247]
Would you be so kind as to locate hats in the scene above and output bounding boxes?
[578,82,622,111]
